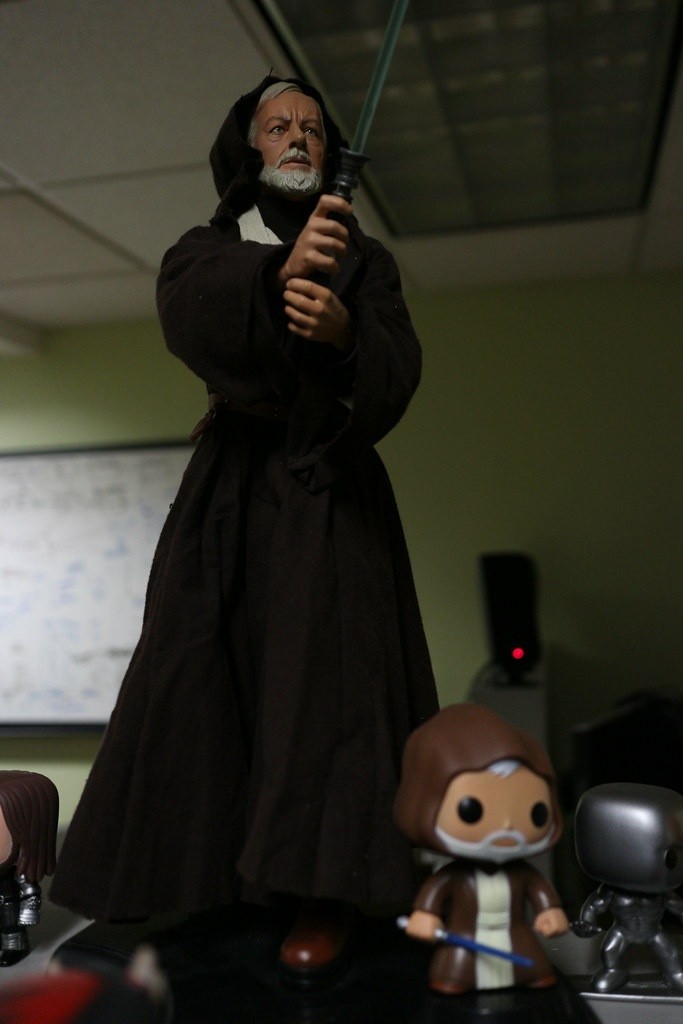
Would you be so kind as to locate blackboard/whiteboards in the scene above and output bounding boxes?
[0,438,199,734]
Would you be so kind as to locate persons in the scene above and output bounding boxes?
[47,74,442,994]
[0,771,59,966]
[395,700,683,1023]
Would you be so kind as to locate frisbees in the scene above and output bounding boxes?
[0,963,176,1024]
